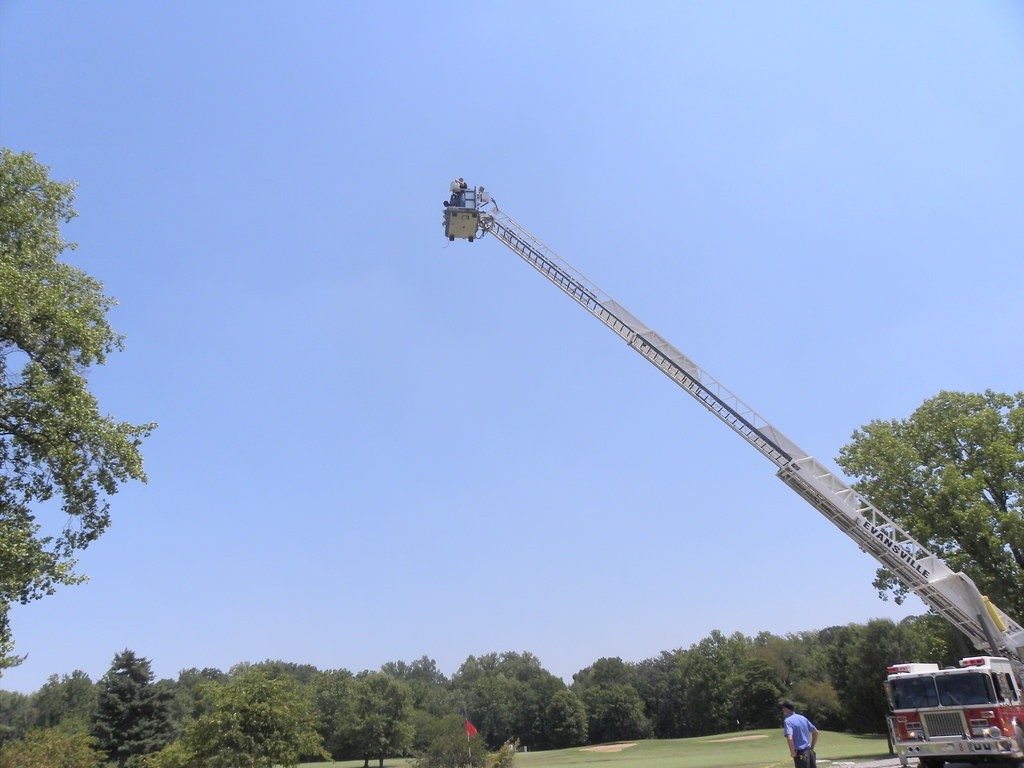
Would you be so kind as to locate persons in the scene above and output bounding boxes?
[443,201,450,207]
[782,700,819,768]
[455,177,468,189]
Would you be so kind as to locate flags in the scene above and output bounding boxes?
[465,719,478,739]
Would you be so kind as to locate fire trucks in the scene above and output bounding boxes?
[444,175,1024,767]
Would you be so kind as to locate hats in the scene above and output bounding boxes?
[778,699,795,712]
